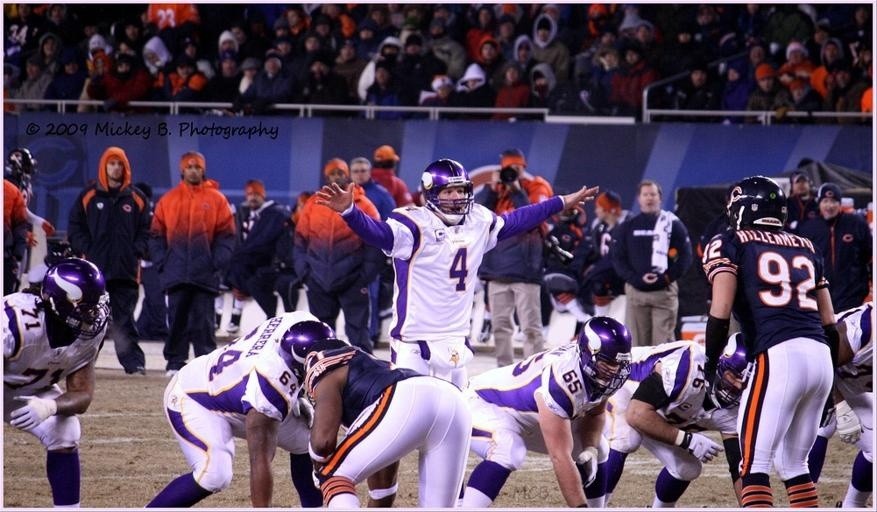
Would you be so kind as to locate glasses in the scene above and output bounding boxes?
[351,168,372,173]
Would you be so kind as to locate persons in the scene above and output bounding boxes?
[3,257,112,508]
[144,308,337,508]
[315,157,600,509]
[301,339,473,509]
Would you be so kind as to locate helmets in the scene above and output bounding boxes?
[278,320,350,378]
[704,330,755,410]
[725,174,788,231]
[420,156,474,226]
[8,146,39,175]
[577,316,633,392]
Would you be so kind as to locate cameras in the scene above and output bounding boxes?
[501,167,517,183]
[546,234,573,265]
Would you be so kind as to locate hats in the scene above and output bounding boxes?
[26,53,45,71]
[595,190,623,217]
[754,64,774,82]
[174,4,454,93]
[373,144,400,163]
[180,152,207,176]
[322,156,350,180]
[244,181,266,200]
[815,182,842,207]
[790,170,811,186]
[500,146,526,171]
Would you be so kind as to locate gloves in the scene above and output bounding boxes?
[20,231,40,248]
[833,399,864,448]
[576,445,599,488]
[33,215,55,237]
[9,394,58,432]
[674,426,724,465]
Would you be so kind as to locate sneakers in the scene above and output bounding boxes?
[127,361,189,376]
